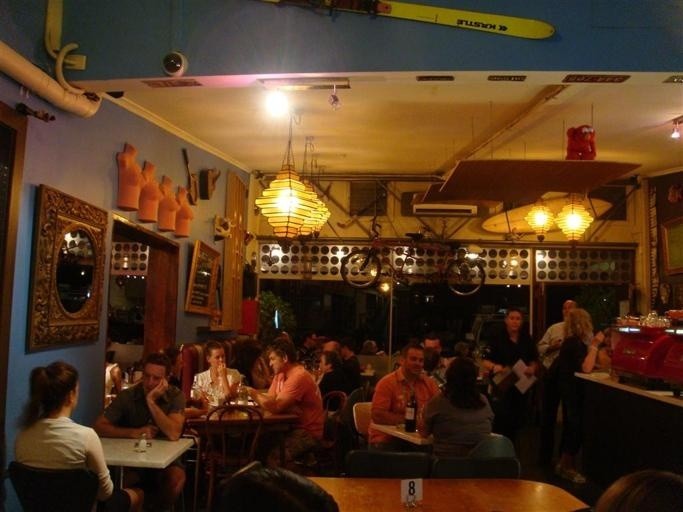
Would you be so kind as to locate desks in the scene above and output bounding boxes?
[358,353,494,387]
[100,434,193,490]
[373,419,435,445]
[184,402,298,467]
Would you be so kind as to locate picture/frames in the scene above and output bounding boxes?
[182,239,222,317]
[659,214,682,276]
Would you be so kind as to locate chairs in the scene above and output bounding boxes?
[466,432,514,459]
[345,448,432,478]
[8,461,99,512]
[312,383,373,470]
[431,455,522,479]
[180,348,211,401]
[219,467,340,512]
[181,404,271,510]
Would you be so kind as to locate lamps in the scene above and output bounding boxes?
[255,115,331,249]
[553,191,595,248]
[524,197,555,242]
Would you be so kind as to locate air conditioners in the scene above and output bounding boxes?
[412,194,477,217]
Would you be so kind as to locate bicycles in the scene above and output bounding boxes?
[335,214,487,299]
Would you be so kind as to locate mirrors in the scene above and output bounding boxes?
[24,184,109,354]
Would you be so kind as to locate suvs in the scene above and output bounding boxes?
[466,311,531,380]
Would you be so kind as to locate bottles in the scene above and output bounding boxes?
[189,376,203,399]
[235,375,248,416]
[404,389,416,431]
[137,432,147,453]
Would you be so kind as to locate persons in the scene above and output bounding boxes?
[483,300,608,481]
[14,362,144,512]
[95,351,186,512]
[366,333,495,477]
[190,330,361,468]
[104,350,122,395]
